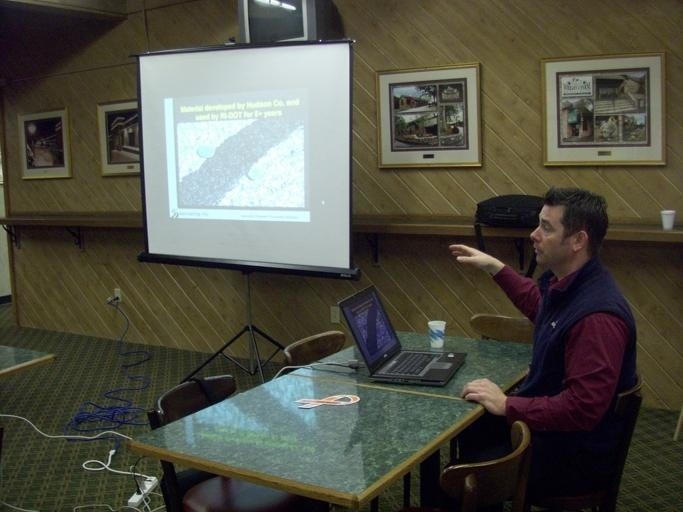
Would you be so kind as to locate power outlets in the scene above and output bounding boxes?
[114,288,122,303]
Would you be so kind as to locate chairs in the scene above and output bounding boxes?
[147,376,302,511]
[435,422,530,508]
[609,363,642,507]
[471,311,533,346]
[284,331,346,369]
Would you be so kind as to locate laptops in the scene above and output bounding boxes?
[336,284,467,387]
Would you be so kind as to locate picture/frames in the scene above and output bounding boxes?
[375,63,481,169]
[99,101,144,178]
[17,108,71,183]
[541,52,667,168]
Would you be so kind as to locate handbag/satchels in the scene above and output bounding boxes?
[475,194,544,230]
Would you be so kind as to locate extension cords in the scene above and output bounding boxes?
[127,476,159,507]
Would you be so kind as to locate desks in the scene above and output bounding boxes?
[126,329,534,510]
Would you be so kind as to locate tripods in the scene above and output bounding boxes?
[181,271,285,383]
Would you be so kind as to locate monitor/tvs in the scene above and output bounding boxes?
[237,0,318,45]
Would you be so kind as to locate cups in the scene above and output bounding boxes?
[659,210,675,231]
[426,319,446,350]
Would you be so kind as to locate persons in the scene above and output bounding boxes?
[447,186,637,512]
